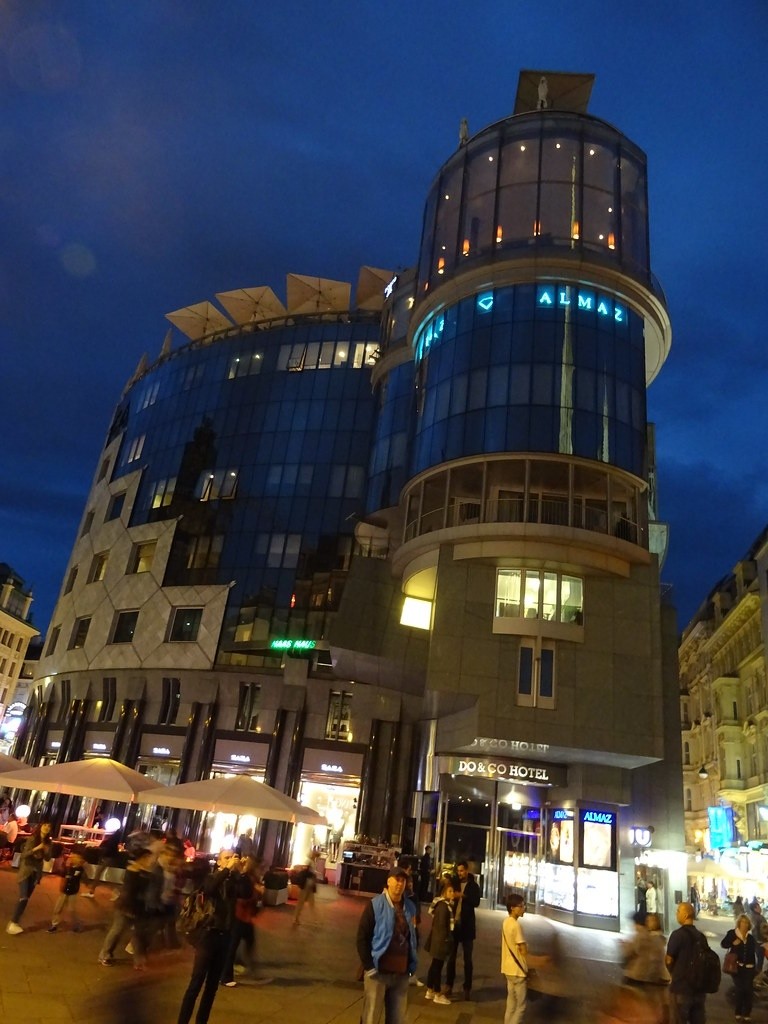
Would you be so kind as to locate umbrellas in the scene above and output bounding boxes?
[0,752,331,851]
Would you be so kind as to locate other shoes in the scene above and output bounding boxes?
[446,986,452,995]
[735,1016,742,1023]
[464,990,470,1000]
[80,893,95,898]
[99,956,113,967]
[743,1018,752,1024]
[125,945,135,955]
[6,922,24,935]
[46,927,57,933]
[219,981,236,987]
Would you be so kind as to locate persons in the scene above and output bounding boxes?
[500,894,549,1024]
[356,846,480,1024]
[0,798,319,1024]
[616,872,768,1024]
[314,807,346,864]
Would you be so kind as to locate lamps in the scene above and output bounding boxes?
[629,825,654,849]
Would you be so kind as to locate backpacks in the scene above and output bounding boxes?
[681,926,721,993]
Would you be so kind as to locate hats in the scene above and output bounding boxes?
[388,866,408,880]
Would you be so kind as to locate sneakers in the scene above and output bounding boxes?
[425,991,437,1000]
[433,994,451,1005]
[409,975,425,987]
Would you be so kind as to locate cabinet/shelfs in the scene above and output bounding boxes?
[343,842,402,868]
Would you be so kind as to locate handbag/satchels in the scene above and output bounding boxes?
[722,954,738,974]
[526,968,542,1000]
